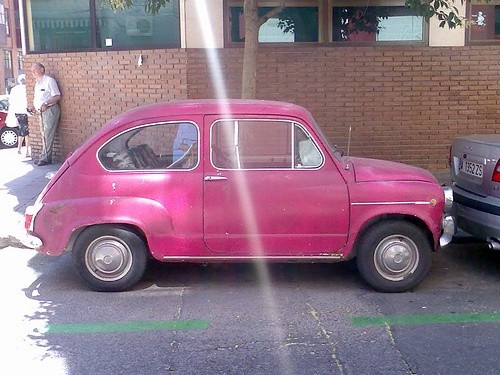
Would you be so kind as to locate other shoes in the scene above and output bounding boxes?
[34,158,49,167]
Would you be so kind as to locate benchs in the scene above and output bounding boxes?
[122,144,161,170]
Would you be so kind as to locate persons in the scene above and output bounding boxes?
[11,74,31,157]
[27,62,62,165]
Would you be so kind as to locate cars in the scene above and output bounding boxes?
[443,135,500,252]
[0,111,25,149]
[25,99,459,295]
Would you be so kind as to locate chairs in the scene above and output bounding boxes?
[213,145,230,168]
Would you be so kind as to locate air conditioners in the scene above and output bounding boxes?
[125,16,152,36]
